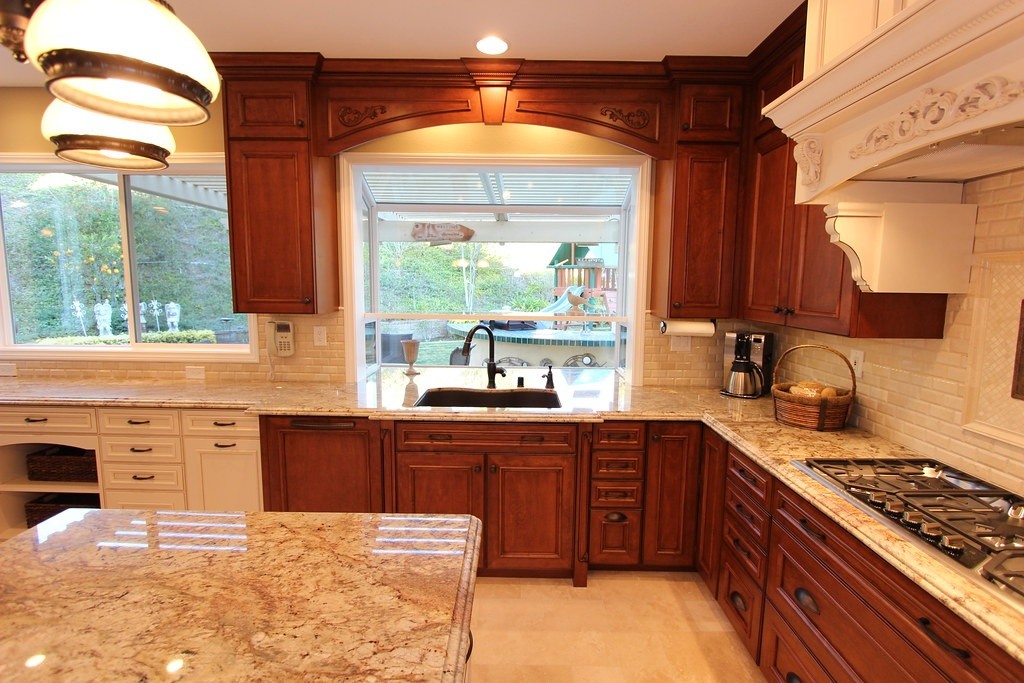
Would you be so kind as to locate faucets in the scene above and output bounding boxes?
[461,324,507,389]
[541,365,555,390]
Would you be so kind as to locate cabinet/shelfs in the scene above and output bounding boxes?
[736,0,948,339]
[219,51,339,315]
[759,1,1024,205]
[259,416,387,513]
[649,56,748,318]
[387,420,583,588]
[696,422,728,599]
[717,443,774,665]
[641,422,702,571]
[757,479,1023,683]
[0,405,262,542]
[583,421,647,587]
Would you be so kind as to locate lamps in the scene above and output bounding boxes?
[0,0,221,171]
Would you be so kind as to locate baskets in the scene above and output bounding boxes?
[771,344,858,432]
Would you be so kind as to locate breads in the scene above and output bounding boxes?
[788,381,836,397]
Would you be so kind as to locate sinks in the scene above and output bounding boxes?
[415,391,561,408]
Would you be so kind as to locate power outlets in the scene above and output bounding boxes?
[848,349,864,377]
[314,326,327,347]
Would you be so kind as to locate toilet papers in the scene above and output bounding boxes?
[663,321,715,338]
